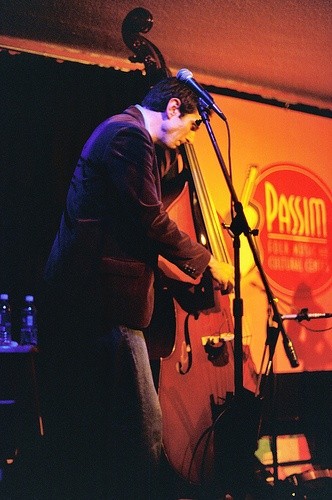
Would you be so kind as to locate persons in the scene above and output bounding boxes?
[37,76,236,500]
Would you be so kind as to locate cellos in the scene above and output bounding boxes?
[121,7,259,490]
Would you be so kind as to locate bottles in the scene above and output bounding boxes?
[0,294,10,346]
[23,295,38,344]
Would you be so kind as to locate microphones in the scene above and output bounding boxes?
[272,312,332,322]
[178,68,226,121]
